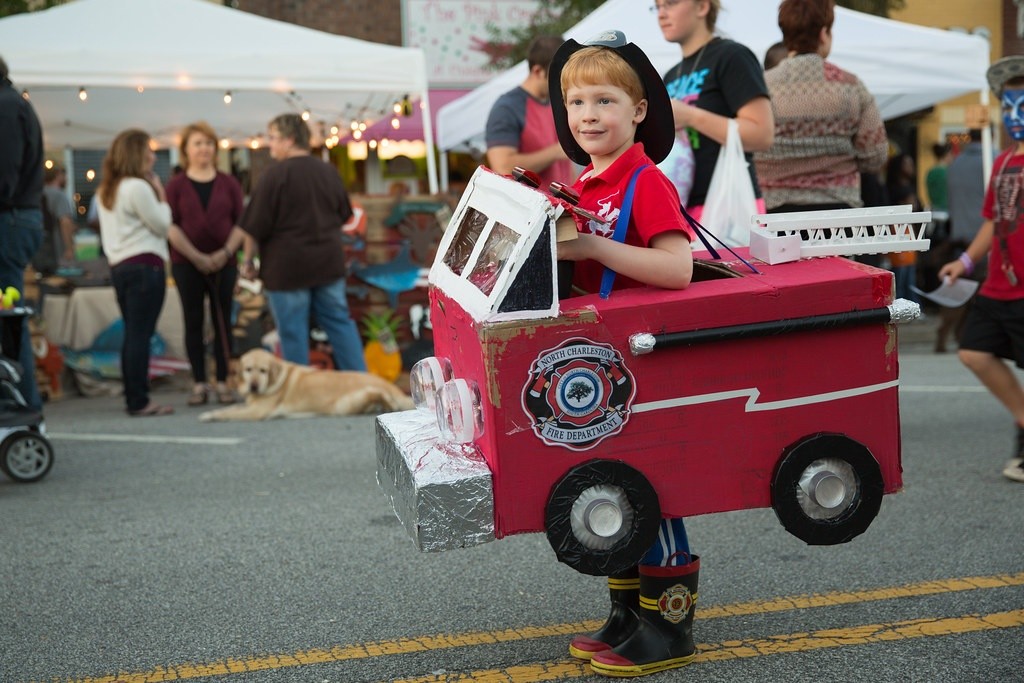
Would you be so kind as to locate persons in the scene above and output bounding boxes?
[483,33,574,202]
[40,158,79,265]
[644,0,774,251]
[86,129,172,417]
[855,137,924,312]
[211,113,367,372]
[938,55,1024,482]
[164,124,245,407]
[0,54,45,442]
[746,0,888,264]
[551,44,701,677]
[925,126,988,350]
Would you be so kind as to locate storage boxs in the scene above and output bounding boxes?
[343,194,460,348]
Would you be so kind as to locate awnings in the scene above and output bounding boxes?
[340,88,471,158]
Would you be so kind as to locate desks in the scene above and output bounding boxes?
[44,286,212,382]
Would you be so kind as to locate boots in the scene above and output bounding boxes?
[590,550,701,676]
[567,568,642,659]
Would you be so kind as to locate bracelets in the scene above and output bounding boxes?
[239,258,256,263]
[223,244,233,260]
[959,252,974,276]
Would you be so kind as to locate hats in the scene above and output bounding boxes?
[548,28,674,166]
[988,54,1024,101]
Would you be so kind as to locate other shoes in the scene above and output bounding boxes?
[189,383,208,407]
[1001,424,1024,484]
[216,381,238,405]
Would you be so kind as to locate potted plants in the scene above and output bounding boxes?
[359,307,406,382]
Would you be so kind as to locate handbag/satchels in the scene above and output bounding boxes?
[693,117,759,250]
[655,111,696,209]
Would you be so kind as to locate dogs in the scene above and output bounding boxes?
[198,349,418,423]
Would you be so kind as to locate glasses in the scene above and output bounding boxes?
[649,0,682,15]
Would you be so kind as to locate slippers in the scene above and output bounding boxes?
[129,402,177,416]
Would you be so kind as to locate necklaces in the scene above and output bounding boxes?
[994,145,1022,285]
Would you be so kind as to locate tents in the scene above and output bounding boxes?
[435,0,992,193]
[0,0,436,196]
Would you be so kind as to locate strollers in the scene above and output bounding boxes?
[0,283,54,483]
